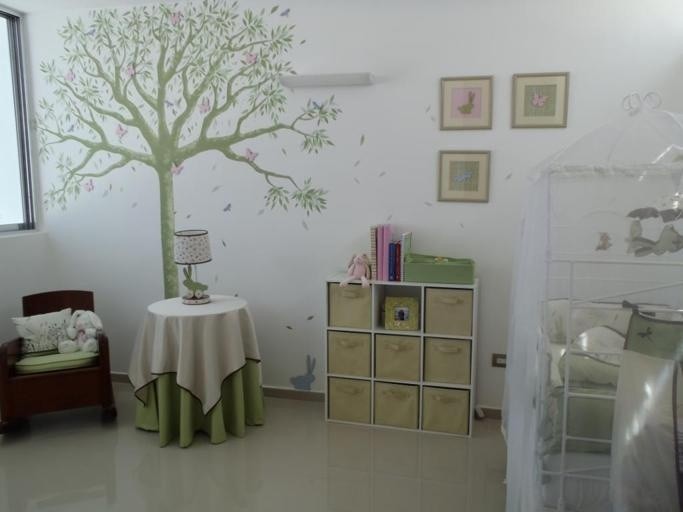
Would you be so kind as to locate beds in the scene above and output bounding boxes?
[528,90,681,511]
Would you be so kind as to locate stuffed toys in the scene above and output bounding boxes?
[58,310,103,354]
[339,253,371,289]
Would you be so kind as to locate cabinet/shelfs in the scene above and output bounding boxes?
[321,264,481,441]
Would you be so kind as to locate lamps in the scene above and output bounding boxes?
[280,68,377,87]
[172,228,213,305]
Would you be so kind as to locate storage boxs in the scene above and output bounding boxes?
[402,253,474,286]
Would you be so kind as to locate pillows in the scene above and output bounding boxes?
[15,348,98,374]
[9,308,75,358]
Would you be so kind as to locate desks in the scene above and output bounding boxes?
[125,293,267,448]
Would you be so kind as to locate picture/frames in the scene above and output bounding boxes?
[437,74,494,133]
[437,147,492,204]
[382,296,420,332]
[509,71,570,129]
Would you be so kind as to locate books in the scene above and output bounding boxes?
[370,224,411,282]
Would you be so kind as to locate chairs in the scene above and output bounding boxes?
[2,286,121,437]
